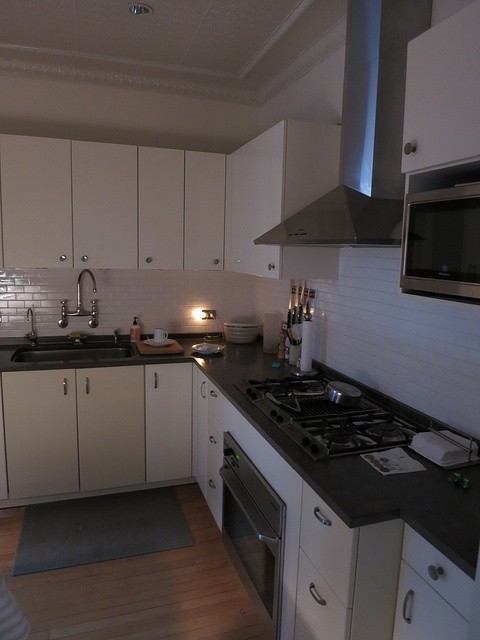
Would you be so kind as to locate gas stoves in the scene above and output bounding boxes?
[232,372,423,462]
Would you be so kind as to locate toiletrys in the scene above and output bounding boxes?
[130,316,141,343]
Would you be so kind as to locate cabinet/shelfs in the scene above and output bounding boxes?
[144,361,194,483]
[194,360,226,532]
[293,481,402,640]
[0,359,147,502]
[392,519,479,640]
[226,118,341,280]
[1,133,226,271]
[398,0,479,175]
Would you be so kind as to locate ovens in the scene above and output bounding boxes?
[219,430,287,639]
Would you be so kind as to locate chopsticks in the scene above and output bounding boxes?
[284,330,302,345]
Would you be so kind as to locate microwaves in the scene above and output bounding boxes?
[399,180,480,300]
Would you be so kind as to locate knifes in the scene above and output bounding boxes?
[287,287,292,328]
[292,283,299,325]
[298,281,305,324]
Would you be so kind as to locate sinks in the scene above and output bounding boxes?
[9,341,136,362]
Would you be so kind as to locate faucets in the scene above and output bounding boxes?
[76,269,97,312]
[26,307,35,335]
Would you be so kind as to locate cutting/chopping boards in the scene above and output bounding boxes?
[136,339,184,355]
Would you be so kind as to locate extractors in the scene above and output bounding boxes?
[253,184,402,249]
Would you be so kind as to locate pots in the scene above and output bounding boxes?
[286,381,362,408]
[223,322,260,344]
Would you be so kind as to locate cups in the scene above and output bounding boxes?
[263,312,280,353]
[154,328,168,343]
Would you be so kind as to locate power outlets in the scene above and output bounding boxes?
[201,309,216,320]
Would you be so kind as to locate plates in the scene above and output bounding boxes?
[191,343,226,354]
[144,339,176,346]
